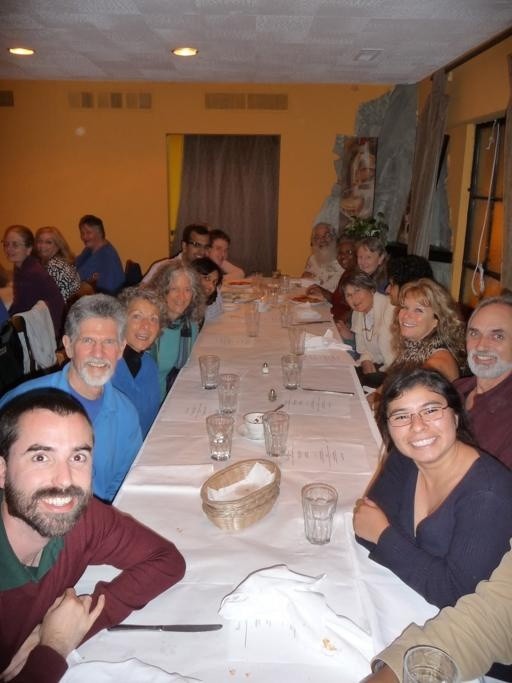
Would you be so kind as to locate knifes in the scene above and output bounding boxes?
[106,623,223,632]
[302,388,356,397]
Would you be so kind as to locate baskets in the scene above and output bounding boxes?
[200,457,282,531]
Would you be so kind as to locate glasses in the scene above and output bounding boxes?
[186,241,211,249]
[3,243,27,250]
[312,233,336,241]
[386,400,449,428]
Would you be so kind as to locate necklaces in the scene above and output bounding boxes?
[364,310,375,341]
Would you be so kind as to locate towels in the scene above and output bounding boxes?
[299,279,321,288]
[293,300,322,320]
[58,463,319,655]
[219,563,384,674]
[303,321,353,357]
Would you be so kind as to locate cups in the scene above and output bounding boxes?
[217,373,240,414]
[280,354,303,391]
[198,354,220,390]
[301,482,338,545]
[262,410,290,457]
[242,411,264,435]
[288,325,306,354]
[245,309,260,336]
[279,304,295,328]
[206,414,235,461]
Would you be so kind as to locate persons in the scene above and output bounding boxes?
[366,277,464,418]
[301,222,340,280]
[73,214,124,294]
[352,373,512,607]
[337,236,390,343]
[189,257,223,319]
[0,293,142,504]
[140,223,210,292]
[1,386,185,682]
[308,236,358,325]
[442,295,511,470]
[209,228,245,282]
[154,261,199,405]
[112,285,162,442]
[34,227,80,300]
[344,272,395,372]
[385,254,433,306]
[361,530,512,682]
[4,223,64,337]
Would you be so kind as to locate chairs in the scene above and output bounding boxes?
[76,281,95,301]
[9,302,55,377]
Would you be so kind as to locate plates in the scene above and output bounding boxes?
[222,302,240,311]
[223,278,254,289]
[285,294,325,307]
[234,422,265,443]
[260,270,291,309]
